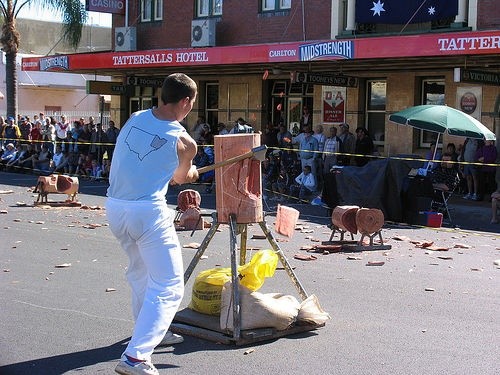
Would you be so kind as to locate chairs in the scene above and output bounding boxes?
[428,168,461,226]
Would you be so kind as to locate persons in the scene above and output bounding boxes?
[105,70,200,375]
[0,113,499,222]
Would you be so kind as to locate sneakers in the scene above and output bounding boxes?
[114,354,158,375]
[156,332,184,345]
[462,192,471,200]
[470,193,481,201]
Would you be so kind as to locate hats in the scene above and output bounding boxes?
[339,123,349,129]
[354,126,369,134]
[7,117,13,121]
[20,116,27,121]
[6,143,15,149]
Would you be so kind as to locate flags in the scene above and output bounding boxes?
[354,0,458,26]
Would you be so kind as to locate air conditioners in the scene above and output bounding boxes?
[191,18,217,48]
[114,26,137,52]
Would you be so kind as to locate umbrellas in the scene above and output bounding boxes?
[389,101,497,172]
